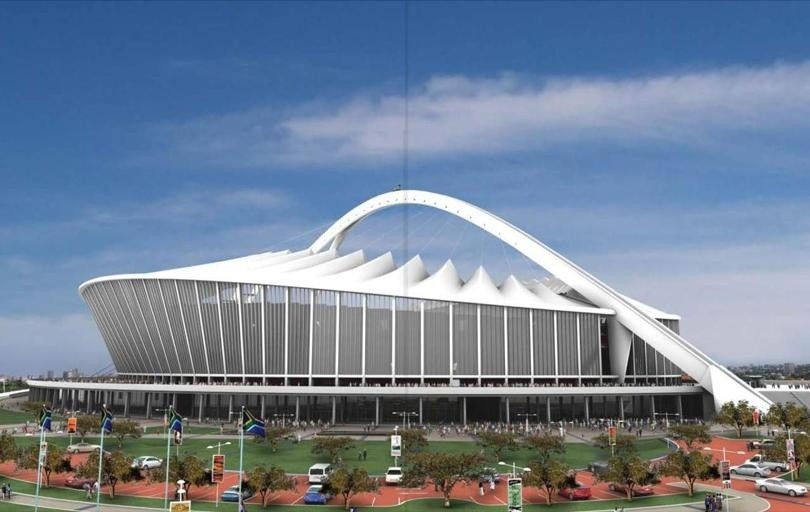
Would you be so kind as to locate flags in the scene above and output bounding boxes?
[101,408,113,432]
[243,408,266,438]
[39,407,52,430]
[169,407,183,434]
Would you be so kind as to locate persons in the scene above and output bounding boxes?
[479,473,496,496]
[83,480,98,500]
[349,507,358,512]
[614,505,625,512]
[767,429,775,436]
[51,407,95,418]
[1,482,12,500]
[360,421,375,432]
[2,421,38,437]
[187,416,224,434]
[265,417,330,432]
[232,419,238,431]
[357,448,368,460]
[704,493,723,512]
[414,415,725,439]
[239,503,246,512]
[746,439,753,452]
